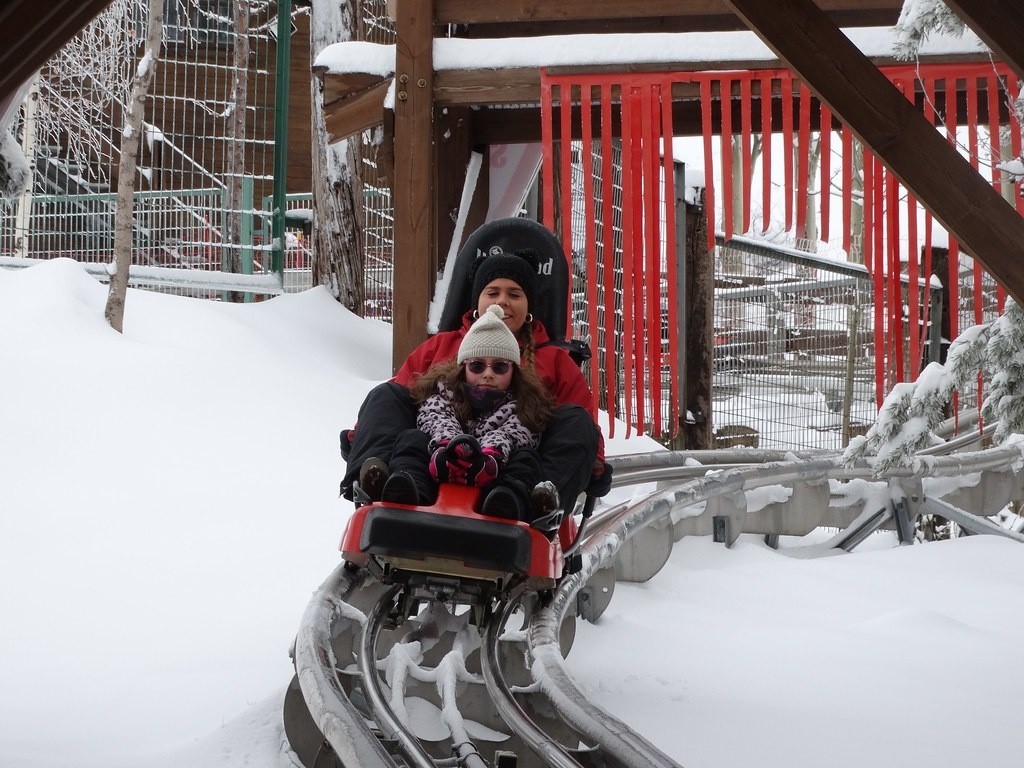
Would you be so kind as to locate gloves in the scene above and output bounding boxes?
[340,429,351,463]
[584,463,613,497]
[429,440,464,483]
[456,446,505,487]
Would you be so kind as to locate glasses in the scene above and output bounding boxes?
[463,358,513,375]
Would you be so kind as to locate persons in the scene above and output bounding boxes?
[339,253,613,566]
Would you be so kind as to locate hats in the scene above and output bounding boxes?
[469,248,536,310]
[457,304,520,366]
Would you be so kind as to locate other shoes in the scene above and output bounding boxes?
[360,456,390,502]
[483,486,520,520]
[381,472,419,505]
[529,481,562,542]
